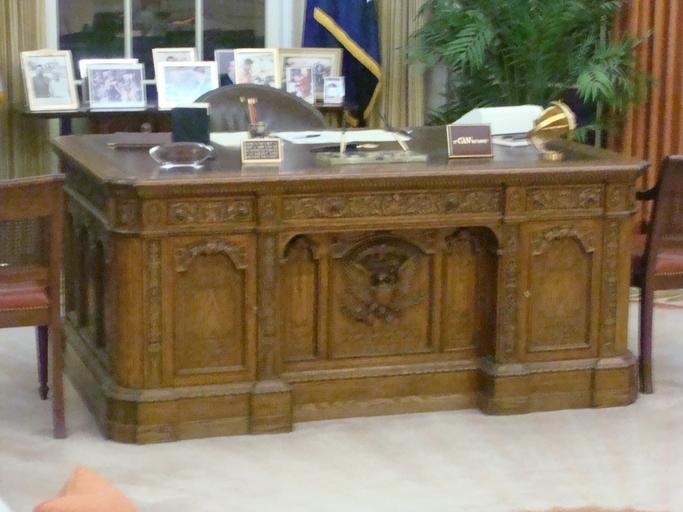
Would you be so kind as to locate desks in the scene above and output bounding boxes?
[49,130,652,442]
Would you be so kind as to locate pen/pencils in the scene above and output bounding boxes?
[306,135,320,137]
[106,143,159,149]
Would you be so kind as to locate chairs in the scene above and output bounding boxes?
[0,174,66,440]
[630,153,681,394]
[197,83,328,130]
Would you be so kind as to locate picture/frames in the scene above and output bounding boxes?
[214,45,345,113]
[19,47,80,112]
[150,45,220,112]
[77,56,148,111]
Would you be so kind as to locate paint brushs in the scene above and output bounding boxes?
[310,143,380,153]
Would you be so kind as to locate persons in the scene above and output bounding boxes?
[32,55,311,103]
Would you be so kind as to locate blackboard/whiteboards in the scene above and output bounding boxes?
[240,138,282,164]
